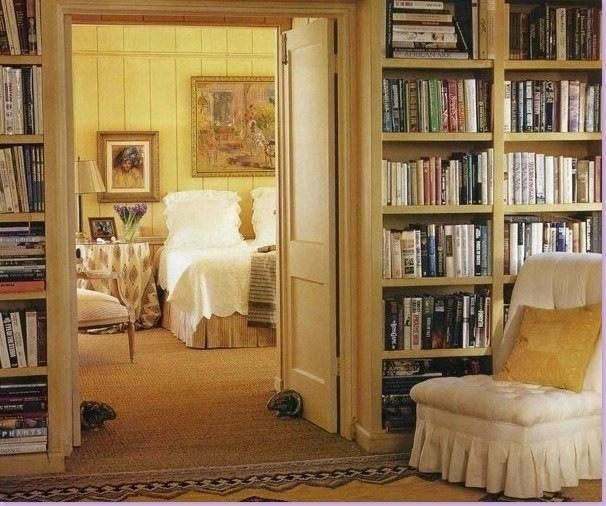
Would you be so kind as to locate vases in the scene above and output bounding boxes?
[119,222,142,243]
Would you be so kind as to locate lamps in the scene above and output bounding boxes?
[71,153,105,239]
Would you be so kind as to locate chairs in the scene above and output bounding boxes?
[67,269,134,364]
[407,249,604,500]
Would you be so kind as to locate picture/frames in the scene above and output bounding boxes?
[86,215,116,240]
[188,74,276,178]
[94,129,159,202]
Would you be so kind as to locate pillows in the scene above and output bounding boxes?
[160,187,241,245]
[494,301,600,392]
[247,184,277,247]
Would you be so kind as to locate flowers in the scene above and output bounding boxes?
[114,201,147,242]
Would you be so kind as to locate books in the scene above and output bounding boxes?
[384,214,596,280]
[383,3,478,60]
[509,2,603,61]
[383,291,511,352]
[383,356,493,431]
[383,73,601,133]
[383,150,604,205]
[0,1,50,457]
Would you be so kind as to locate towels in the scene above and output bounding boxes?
[245,242,277,333]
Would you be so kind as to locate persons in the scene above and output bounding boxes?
[112,148,144,189]
[94,224,109,236]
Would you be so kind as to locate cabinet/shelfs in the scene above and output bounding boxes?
[0,0,78,475]
[357,0,606,454]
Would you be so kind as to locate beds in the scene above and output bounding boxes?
[151,239,280,350]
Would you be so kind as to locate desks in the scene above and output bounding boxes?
[73,240,162,335]
